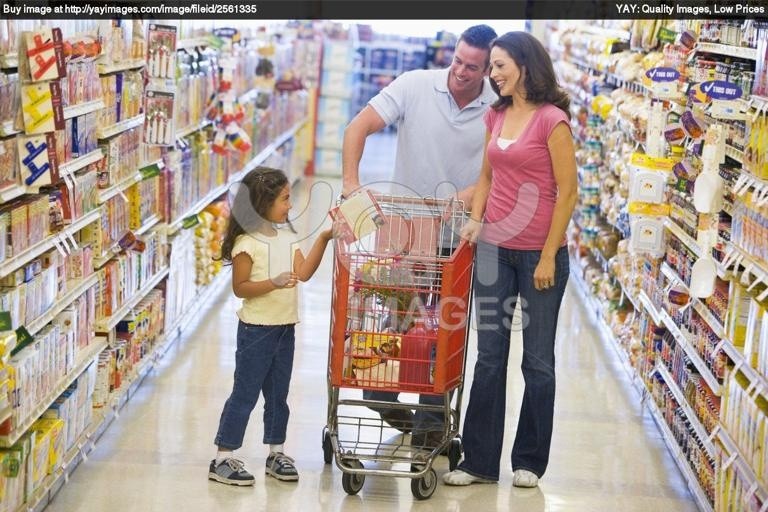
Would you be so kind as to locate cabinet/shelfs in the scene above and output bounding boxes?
[1,20,308,512]
[354,43,427,134]
[567,19,767,512]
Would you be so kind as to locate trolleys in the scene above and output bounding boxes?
[321,190,475,501]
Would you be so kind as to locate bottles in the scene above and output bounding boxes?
[660,306,722,503]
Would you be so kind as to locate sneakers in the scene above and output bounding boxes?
[265,452,299,482]
[208,458,255,486]
[512,470,539,487]
[441,469,496,485]
[411,431,463,454]
[380,400,415,432]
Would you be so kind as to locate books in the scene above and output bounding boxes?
[13,27,67,187]
[328,189,388,246]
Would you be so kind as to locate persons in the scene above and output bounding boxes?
[208,166,346,486]
[342,24,499,455]
[441,31,578,487]
